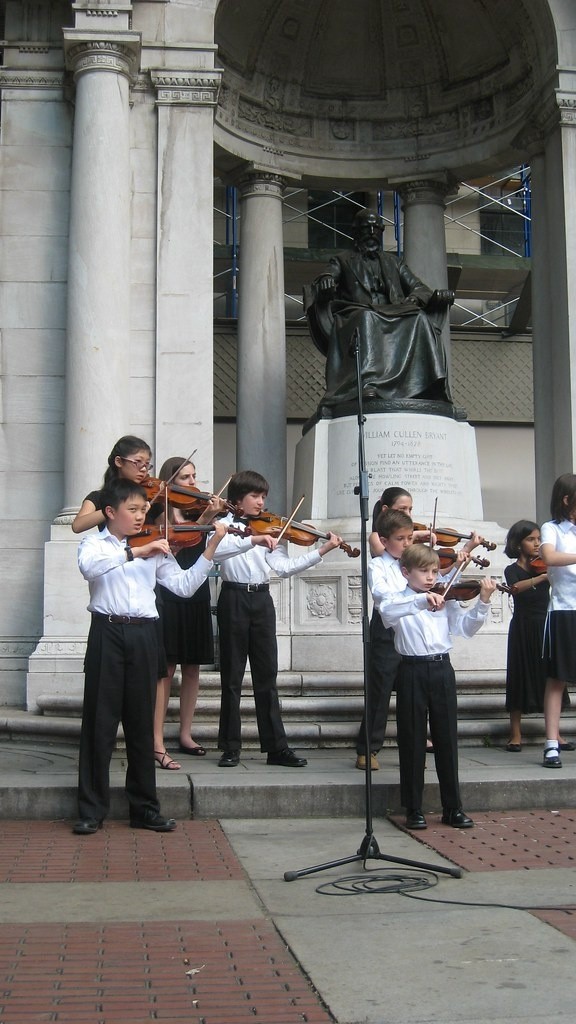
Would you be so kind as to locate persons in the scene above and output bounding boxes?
[538,472,576,768]
[206,470,343,768]
[301,208,457,408]
[504,520,574,752]
[71,477,229,834]
[354,509,469,771]
[72,435,227,770]
[368,487,484,752]
[378,544,496,829]
[155,458,226,755]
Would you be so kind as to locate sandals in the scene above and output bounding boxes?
[154,750,179,769]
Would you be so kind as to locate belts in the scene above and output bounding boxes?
[221,581,269,592]
[401,653,451,662]
[92,612,154,624]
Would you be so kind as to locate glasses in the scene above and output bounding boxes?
[120,456,154,470]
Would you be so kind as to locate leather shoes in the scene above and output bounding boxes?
[441,810,473,826]
[406,808,428,828]
[543,748,562,767]
[219,749,241,766]
[267,748,307,766]
[73,817,103,834]
[180,744,205,754]
[130,808,176,831]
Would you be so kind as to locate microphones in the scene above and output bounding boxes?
[349,327,359,358]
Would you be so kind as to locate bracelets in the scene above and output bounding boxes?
[124,547,134,562]
[531,577,534,590]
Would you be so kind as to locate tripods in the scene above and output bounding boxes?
[282,353,464,882]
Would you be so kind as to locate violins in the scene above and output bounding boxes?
[182,492,231,515]
[435,548,490,570]
[528,557,547,573]
[139,474,245,517]
[127,520,252,560]
[249,510,361,558]
[429,579,519,601]
[412,522,497,552]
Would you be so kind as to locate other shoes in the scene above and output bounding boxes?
[507,740,522,751]
[356,753,378,769]
[558,743,574,751]
